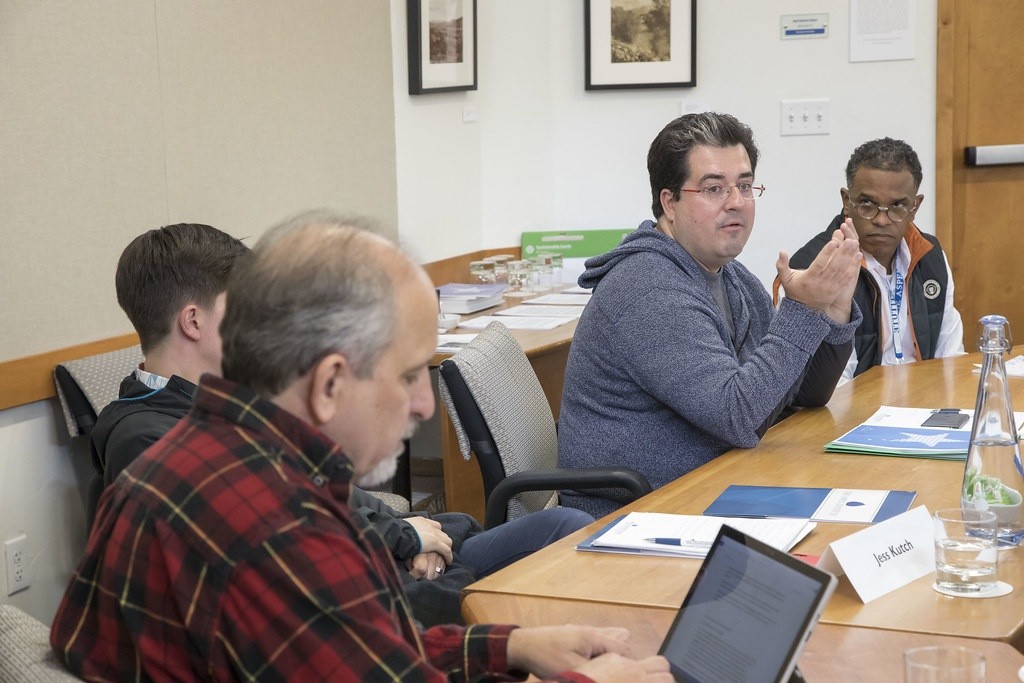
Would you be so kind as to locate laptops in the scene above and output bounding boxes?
[656,523,839,683]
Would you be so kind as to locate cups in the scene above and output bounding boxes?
[508,256,563,293]
[469,260,496,285]
[960,482,1023,523]
[904,646,986,683]
[934,509,997,594]
[482,253,515,284]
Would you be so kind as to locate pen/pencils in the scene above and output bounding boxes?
[930,409,974,414]
[643,538,714,548]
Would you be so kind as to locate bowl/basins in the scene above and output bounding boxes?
[438,314,460,329]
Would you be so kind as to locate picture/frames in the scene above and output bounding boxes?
[585,0,697,90]
[407,0,478,95]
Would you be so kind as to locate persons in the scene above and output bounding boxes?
[50,205,675,683]
[557,112,863,523]
[87,224,595,582]
[772,136,968,385]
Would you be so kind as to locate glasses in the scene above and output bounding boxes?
[846,193,918,222]
[677,183,765,201]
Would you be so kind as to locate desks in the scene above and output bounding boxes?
[459,343,1024,683]
[391,281,594,528]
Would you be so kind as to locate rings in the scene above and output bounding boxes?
[436,567,444,574]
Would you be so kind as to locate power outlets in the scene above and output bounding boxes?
[5,534,31,595]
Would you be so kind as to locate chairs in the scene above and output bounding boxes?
[436,320,654,530]
[0,604,87,683]
[56,339,146,436]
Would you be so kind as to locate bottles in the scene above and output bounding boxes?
[961,314,1024,549]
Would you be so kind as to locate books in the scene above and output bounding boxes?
[573,509,819,561]
[702,484,918,525]
